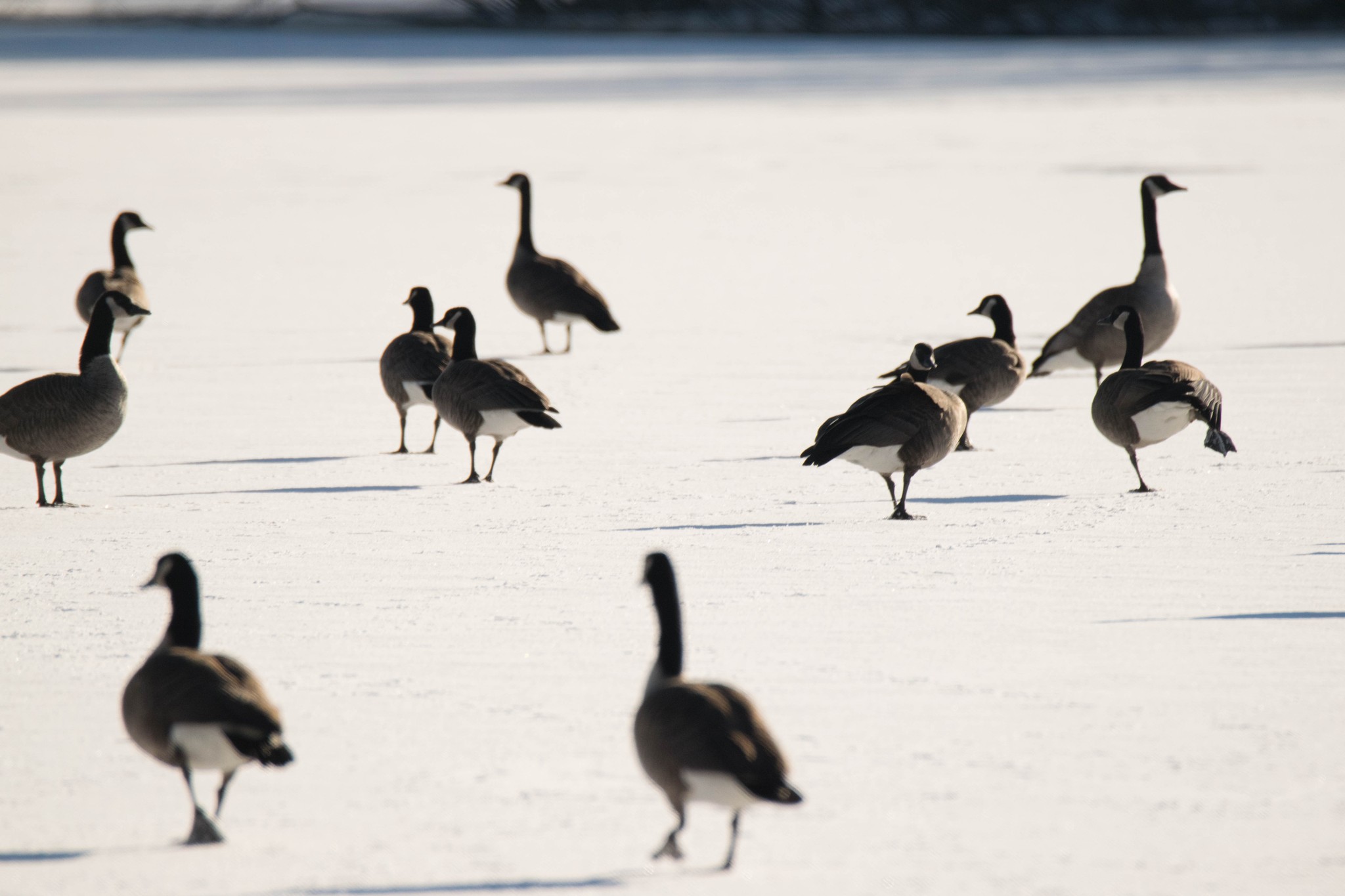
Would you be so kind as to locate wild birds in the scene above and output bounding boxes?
[633,552,802,870]
[76,211,155,362]
[380,286,562,484]
[123,550,295,842]
[497,172,621,353]
[0,290,152,508]
[801,175,1235,520]
